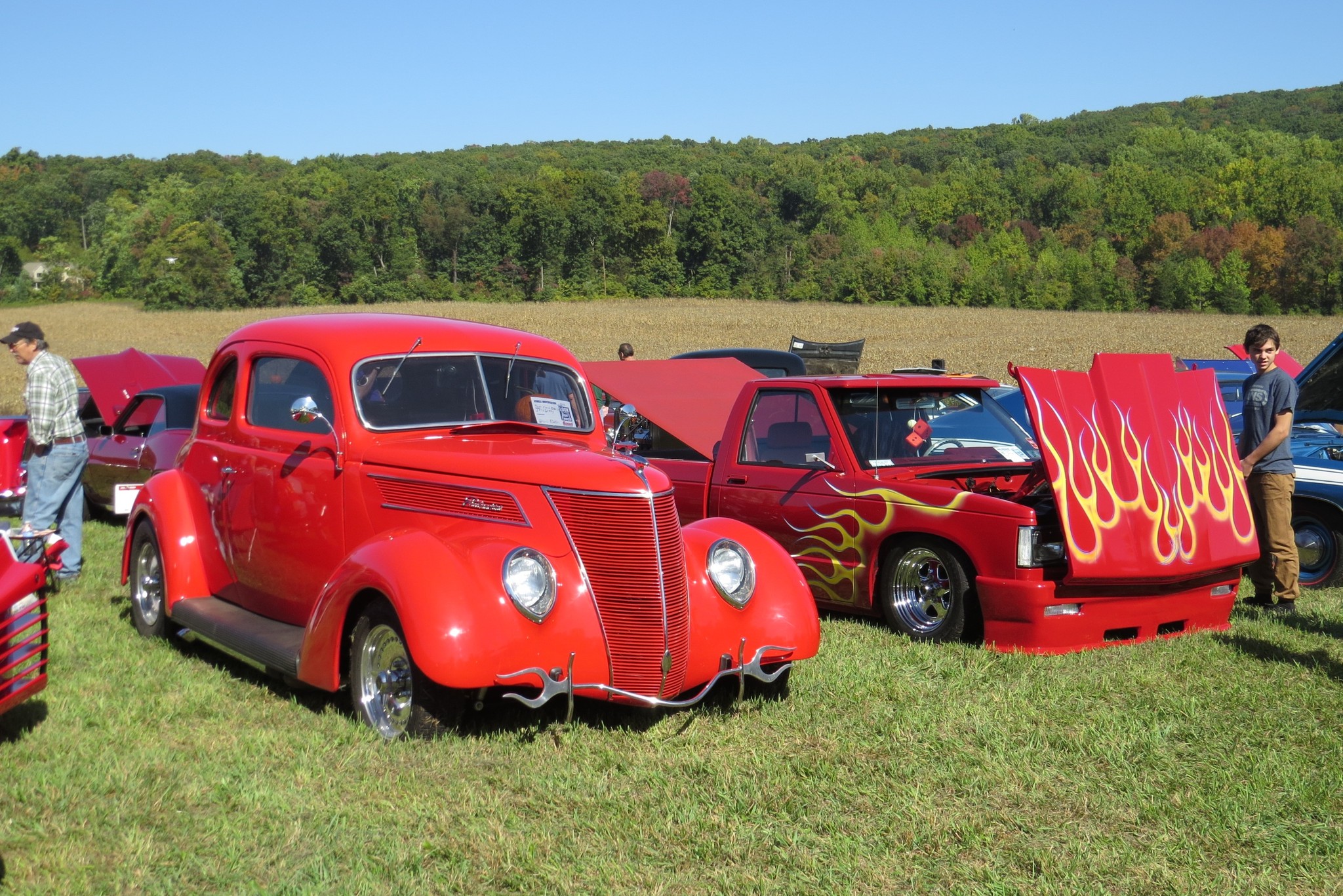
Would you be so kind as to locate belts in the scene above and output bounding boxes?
[55,435,85,445]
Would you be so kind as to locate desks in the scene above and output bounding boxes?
[0,520,69,594]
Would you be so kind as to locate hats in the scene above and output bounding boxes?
[0,322,44,344]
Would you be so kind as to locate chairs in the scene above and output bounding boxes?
[853,417,915,459]
[760,421,825,464]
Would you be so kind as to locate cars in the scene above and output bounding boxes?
[0,412,50,715]
[579,334,1260,655]
[71,348,230,522]
[121,313,821,742]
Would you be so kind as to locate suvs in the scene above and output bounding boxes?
[1175,330,1343,586]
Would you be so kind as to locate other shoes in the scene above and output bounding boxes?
[1265,603,1293,614]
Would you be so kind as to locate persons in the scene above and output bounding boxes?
[1238,323,1300,612]
[536,369,581,427]
[357,366,387,402]
[618,343,651,439]
[0,322,88,582]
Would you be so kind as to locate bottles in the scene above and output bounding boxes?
[22,520,38,556]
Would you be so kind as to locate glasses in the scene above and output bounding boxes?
[8,341,25,349]
